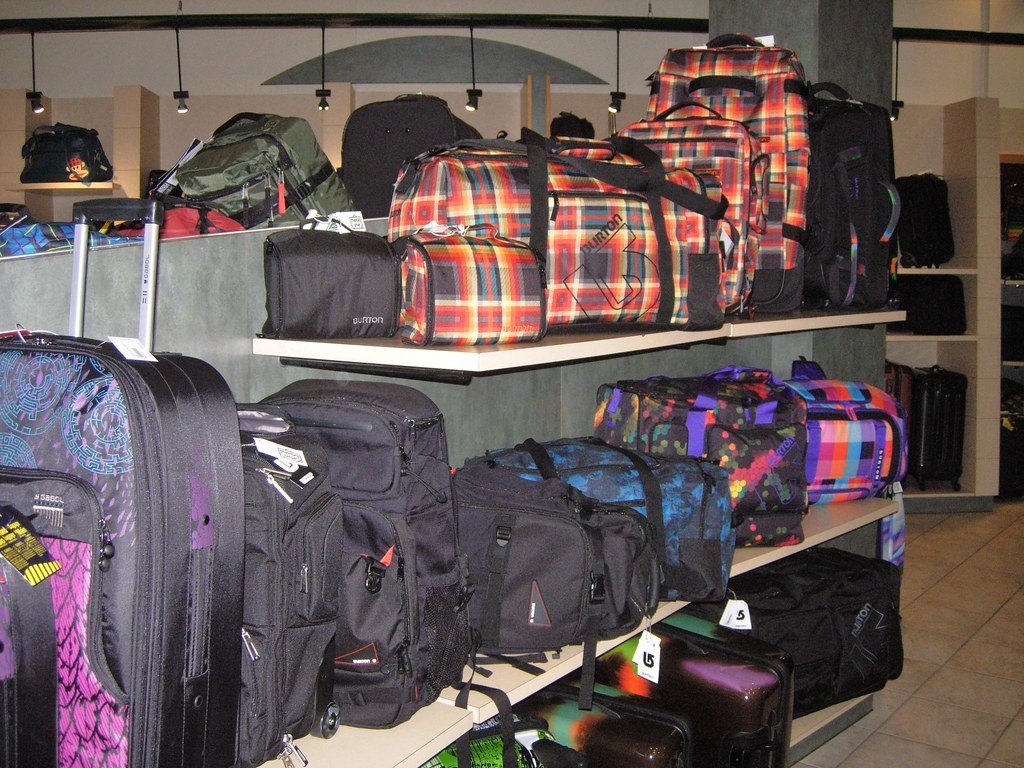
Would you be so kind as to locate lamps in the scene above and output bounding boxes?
[463,23,484,110]
[890,39,906,123]
[25,33,44,114]
[317,27,333,111]
[173,28,190,113]
[606,30,626,113]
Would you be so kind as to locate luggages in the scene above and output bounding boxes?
[0,196,241,768]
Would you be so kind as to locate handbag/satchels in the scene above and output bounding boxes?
[0,29,1024,768]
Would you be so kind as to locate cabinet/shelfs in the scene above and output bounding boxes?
[1002,280,1024,368]
[235,307,904,768]
[885,268,980,497]
